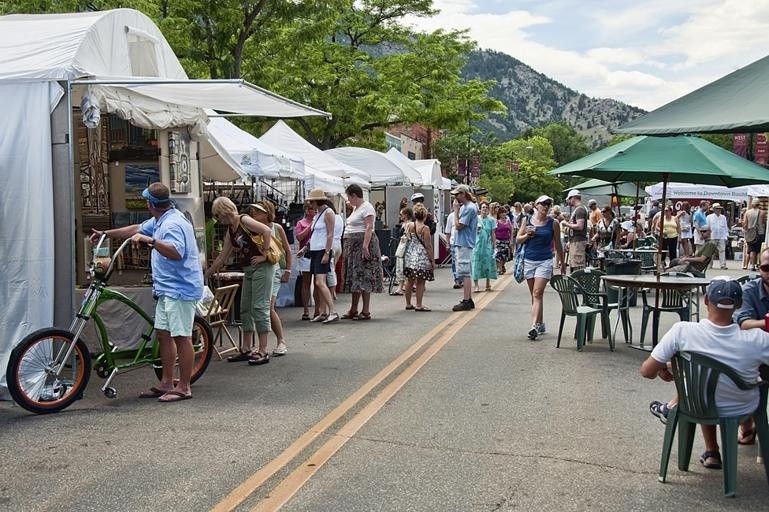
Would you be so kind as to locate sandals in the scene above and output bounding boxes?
[248,353,270,366]
[273,341,288,355]
[650,401,671,425]
[700,451,722,468]
[353,312,371,320]
[227,349,252,362]
[341,311,359,319]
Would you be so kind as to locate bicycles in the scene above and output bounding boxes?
[5,231,214,416]
[388,236,416,293]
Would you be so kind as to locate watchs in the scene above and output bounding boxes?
[148,238,158,247]
[325,251,332,256]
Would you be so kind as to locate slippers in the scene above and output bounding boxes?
[138,387,165,398]
[159,390,192,402]
[739,428,757,444]
[389,291,403,296]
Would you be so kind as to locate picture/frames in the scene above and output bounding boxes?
[195,285,221,317]
[168,130,192,194]
[107,113,160,161]
[170,198,208,272]
[125,165,159,208]
[369,189,387,228]
[84,238,111,272]
[79,117,111,233]
[112,213,153,271]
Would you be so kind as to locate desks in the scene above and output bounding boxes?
[603,249,670,292]
[601,274,712,352]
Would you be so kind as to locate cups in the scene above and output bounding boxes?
[527,226,536,238]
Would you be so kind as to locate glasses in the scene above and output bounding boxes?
[601,210,607,213]
[700,230,710,233]
[514,205,517,207]
[540,202,551,207]
[399,214,404,216]
[665,210,669,211]
[501,210,507,212]
[494,206,500,208]
[310,201,315,204]
[482,207,488,208]
[758,264,769,272]
[215,213,219,219]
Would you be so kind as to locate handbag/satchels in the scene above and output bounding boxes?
[239,215,283,264]
[514,215,531,283]
[680,227,690,232]
[274,224,288,270]
[304,206,330,259]
[395,222,410,258]
[745,209,760,244]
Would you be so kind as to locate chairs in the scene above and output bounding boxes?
[640,272,694,347]
[659,350,769,499]
[635,237,651,248]
[647,235,657,245]
[586,250,598,266]
[637,246,659,275]
[551,274,613,353]
[568,269,628,343]
[608,249,625,259]
[585,251,605,269]
[687,239,719,294]
[737,274,761,285]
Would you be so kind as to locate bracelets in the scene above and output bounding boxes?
[262,248,272,253]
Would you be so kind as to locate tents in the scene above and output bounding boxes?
[318,147,412,239]
[200,109,305,308]
[410,159,451,262]
[385,146,422,232]
[0,10,336,402]
[256,119,369,224]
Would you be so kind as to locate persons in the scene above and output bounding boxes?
[512,202,523,253]
[590,207,622,257]
[637,221,646,239]
[91,183,210,402]
[201,201,218,260]
[692,201,710,253]
[328,201,344,299]
[496,206,513,275]
[247,200,292,353]
[296,201,321,320]
[552,205,562,220]
[677,202,692,258]
[665,225,717,271]
[451,185,478,313]
[489,202,499,219]
[342,185,384,321]
[732,248,769,445]
[445,212,463,288]
[640,277,769,468]
[562,189,588,274]
[706,203,730,269]
[588,199,602,226]
[405,207,435,312]
[621,221,639,250]
[389,207,414,296]
[656,207,681,260]
[204,197,272,365]
[516,196,567,341]
[648,201,661,229]
[524,203,533,215]
[742,198,765,271]
[503,204,514,222]
[473,202,496,292]
[296,189,340,323]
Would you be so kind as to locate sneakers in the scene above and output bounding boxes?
[454,299,475,309]
[310,314,327,322]
[538,323,546,333]
[528,325,538,340]
[751,268,756,271]
[302,314,310,321]
[474,287,480,293]
[452,300,471,311]
[322,313,339,324]
[453,282,463,288]
[415,306,431,311]
[743,267,747,269]
[314,313,320,318]
[406,305,416,310]
[721,266,728,269]
[486,286,491,291]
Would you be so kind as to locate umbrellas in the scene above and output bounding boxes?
[547,137,768,275]
[581,183,653,212]
[611,56,769,136]
[560,179,628,222]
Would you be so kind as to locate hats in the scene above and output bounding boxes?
[534,195,553,206]
[752,198,761,204]
[713,203,723,209]
[411,192,424,200]
[305,188,327,201]
[142,187,169,208]
[707,276,743,308]
[450,184,469,195]
[587,200,596,206]
[565,190,581,201]
[621,221,633,232]
[553,205,561,213]
[249,204,267,213]
